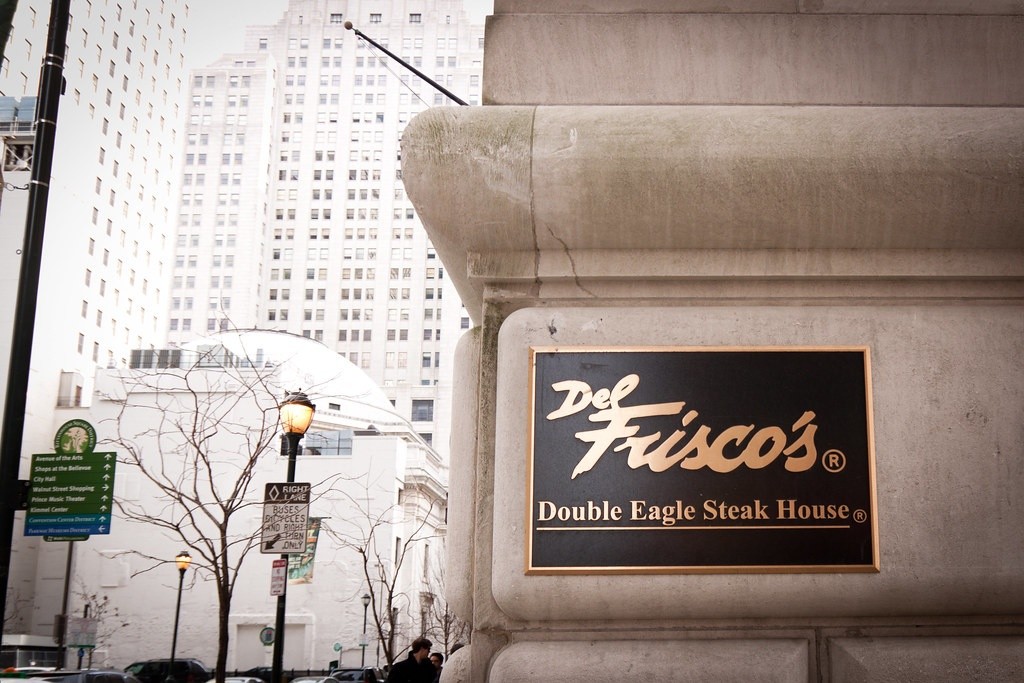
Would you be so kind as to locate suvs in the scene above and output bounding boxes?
[329,665,388,683]
[123,658,209,683]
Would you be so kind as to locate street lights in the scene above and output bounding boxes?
[165,549,193,683]
[361,593,372,668]
[269,391,317,683]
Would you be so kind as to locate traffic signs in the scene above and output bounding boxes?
[259,482,311,555]
[270,558,287,596]
[22,450,117,537]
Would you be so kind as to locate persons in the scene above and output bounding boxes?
[384,638,438,683]
[307,519,319,537]
[429,652,444,683]
[450,644,463,653]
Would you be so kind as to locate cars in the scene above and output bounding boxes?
[289,676,339,683]
[0,666,140,683]
